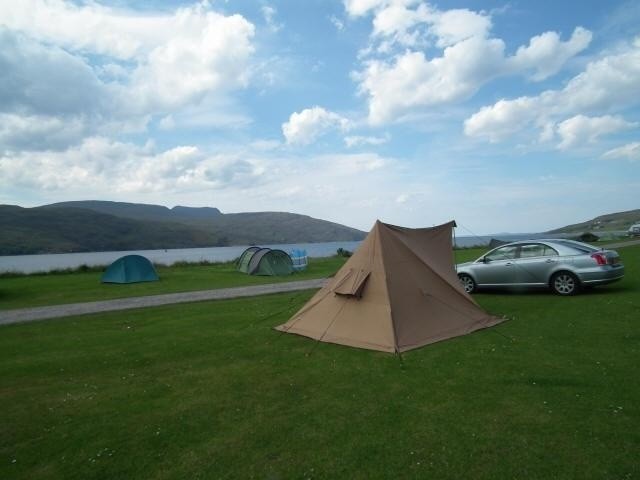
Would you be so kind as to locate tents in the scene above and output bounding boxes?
[235,246,294,277]
[270,219,512,356]
[99,254,161,284]
[487,237,509,250]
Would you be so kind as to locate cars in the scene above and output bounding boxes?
[626,226,640,238]
[453,238,625,296]
